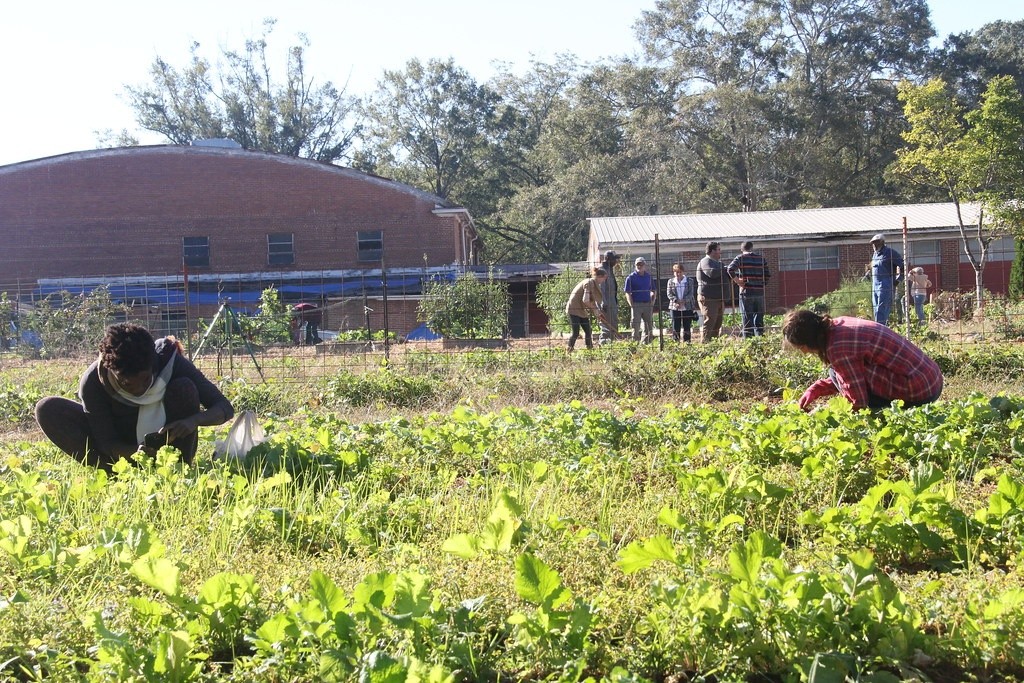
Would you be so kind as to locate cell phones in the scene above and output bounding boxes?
[145,432,166,451]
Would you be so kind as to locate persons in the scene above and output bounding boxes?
[291,303,323,346]
[596,250,623,346]
[666,263,695,344]
[624,257,656,345]
[566,266,608,353]
[783,309,944,416]
[895,266,905,324]
[696,241,731,346]
[726,241,771,340]
[907,267,932,326]
[864,234,904,327]
[35,321,235,482]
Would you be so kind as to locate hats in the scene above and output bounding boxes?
[635,257,646,265]
[913,267,924,273]
[870,234,885,242]
[604,250,623,260]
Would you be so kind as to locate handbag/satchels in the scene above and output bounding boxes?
[212,410,272,463]
